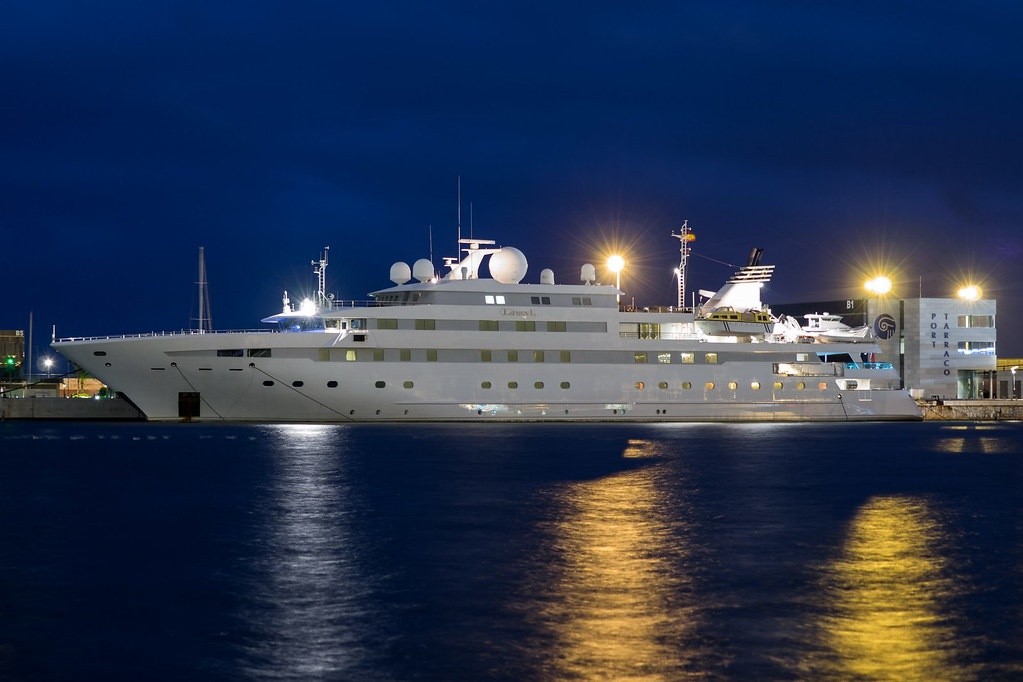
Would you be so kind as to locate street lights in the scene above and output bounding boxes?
[1011,369,1016,400]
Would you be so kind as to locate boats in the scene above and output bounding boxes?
[48,238,924,431]
